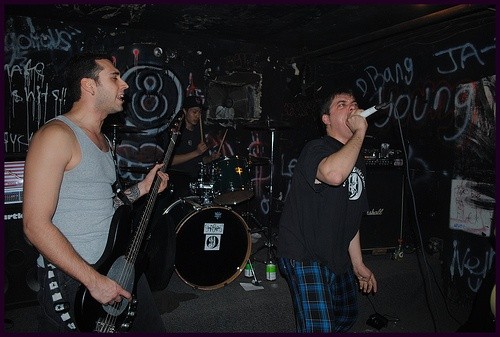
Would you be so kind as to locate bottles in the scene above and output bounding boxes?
[245,256,254,277]
[266,259,276,281]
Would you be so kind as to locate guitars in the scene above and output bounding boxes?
[76,109,188,337]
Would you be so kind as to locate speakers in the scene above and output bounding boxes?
[359,168,405,252]
[5,221,42,310]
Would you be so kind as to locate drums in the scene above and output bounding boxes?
[198,155,254,205]
[162,195,252,291]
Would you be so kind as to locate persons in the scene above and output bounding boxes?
[155,96,209,195]
[279,86,377,332]
[23,52,170,332]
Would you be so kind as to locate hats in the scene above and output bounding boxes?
[184,96,201,109]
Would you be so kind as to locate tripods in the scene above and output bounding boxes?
[240,129,281,285]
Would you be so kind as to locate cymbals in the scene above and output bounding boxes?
[239,120,297,129]
[101,124,142,133]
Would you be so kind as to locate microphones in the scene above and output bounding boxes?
[359,102,389,118]
